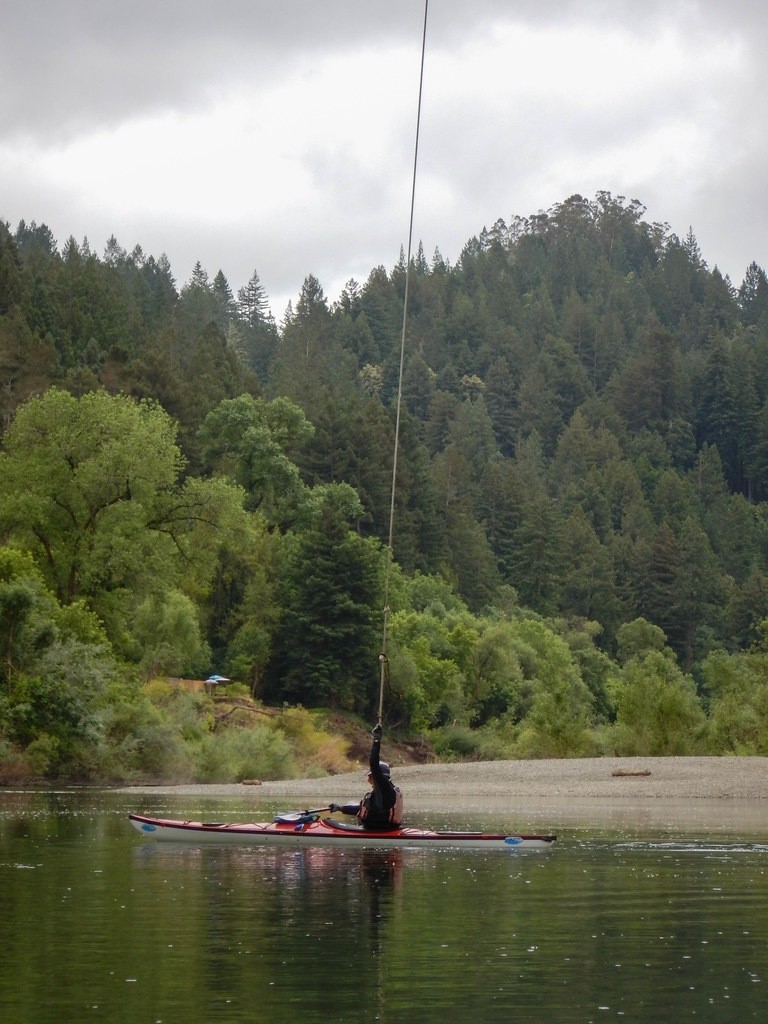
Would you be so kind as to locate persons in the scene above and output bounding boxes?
[328,725,403,830]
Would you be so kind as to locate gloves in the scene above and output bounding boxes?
[329,804,340,813]
[372,725,382,741]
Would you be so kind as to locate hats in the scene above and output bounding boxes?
[368,761,391,778]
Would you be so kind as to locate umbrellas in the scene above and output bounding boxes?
[205,675,230,693]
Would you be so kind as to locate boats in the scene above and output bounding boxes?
[127,812,557,849]
